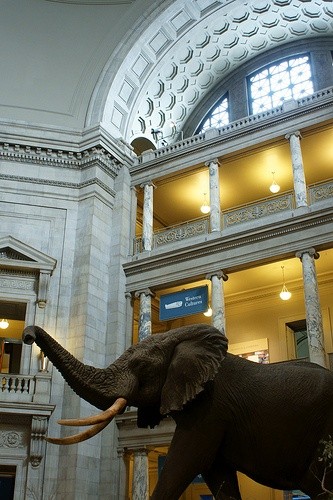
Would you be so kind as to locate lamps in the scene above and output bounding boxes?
[279,265,291,300]
[204,305,213,317]
[270,172,280,193]
[0,318,9,329]
[200,193,210,213]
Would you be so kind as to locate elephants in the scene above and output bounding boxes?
[21,323,333,499]
